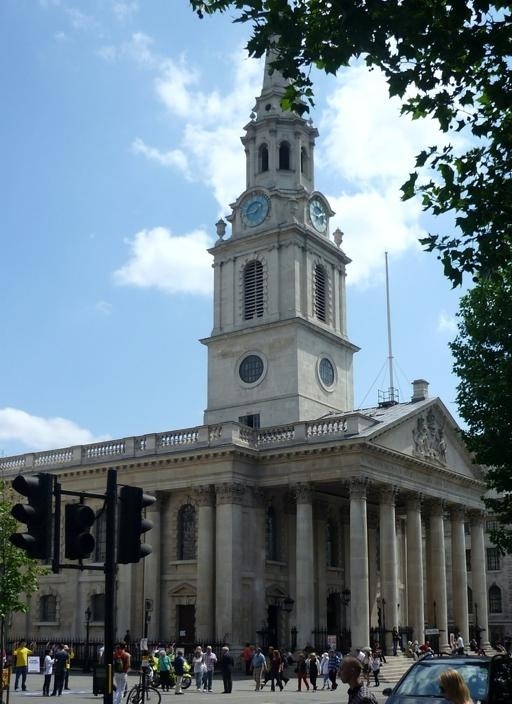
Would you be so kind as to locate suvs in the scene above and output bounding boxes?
[381,651,512,704]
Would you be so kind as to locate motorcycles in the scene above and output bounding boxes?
[146,650,193,691]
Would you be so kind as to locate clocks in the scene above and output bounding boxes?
[239,187,274,229]
[308,196,329,234]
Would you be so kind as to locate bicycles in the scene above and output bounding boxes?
[124,666,162,704]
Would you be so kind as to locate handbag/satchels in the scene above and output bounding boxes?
[183,663,190,672]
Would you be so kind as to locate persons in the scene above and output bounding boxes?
[50,644,69,697]
[62,644,74,690]
[97,627,486,704]
[42,642,57,697]
[12,638,37,692]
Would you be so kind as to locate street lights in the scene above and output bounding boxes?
[84,607,92,672]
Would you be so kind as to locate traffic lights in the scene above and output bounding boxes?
[65,503,98,560]
[117,485,158,564]
[9,474,53,560]
